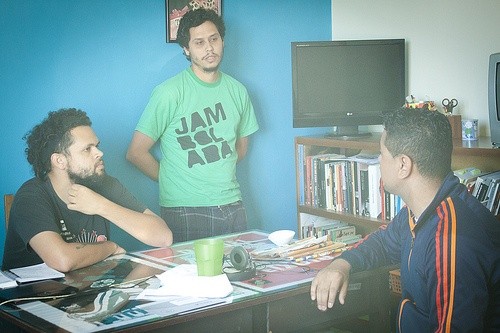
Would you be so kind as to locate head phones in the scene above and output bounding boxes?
[220,246,257,281]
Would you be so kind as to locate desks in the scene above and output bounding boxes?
[0,228,393,333]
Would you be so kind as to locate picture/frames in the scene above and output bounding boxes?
[166,0,222,43]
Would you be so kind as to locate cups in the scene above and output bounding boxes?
[462,119,478,141]
[446,115,462,141]
[269,230,296,247]
[193,238,224,277]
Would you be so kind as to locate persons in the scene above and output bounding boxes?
[3,108,173,272]
[126,8,259,245]
[311,108,500,333]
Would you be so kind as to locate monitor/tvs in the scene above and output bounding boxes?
[290,38,408,140]
[488,52,500,149]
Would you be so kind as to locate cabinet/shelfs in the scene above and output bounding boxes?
[294,136,500,333]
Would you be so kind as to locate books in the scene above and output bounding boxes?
[453,167,500,217]
[0,263,65,289]
[302,150,406,245]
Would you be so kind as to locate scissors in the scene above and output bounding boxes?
[442,98,458,116]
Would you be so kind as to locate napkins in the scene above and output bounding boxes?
[155,264,234,298]
